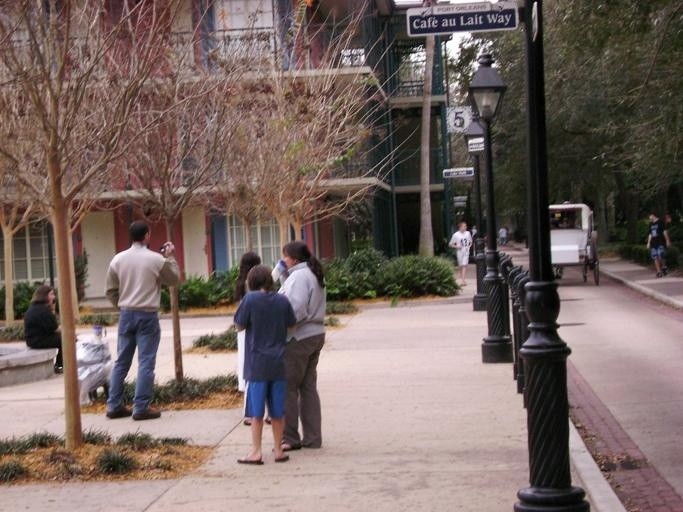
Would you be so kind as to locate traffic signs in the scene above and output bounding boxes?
[442,168,475,178]
[406,5,523,37]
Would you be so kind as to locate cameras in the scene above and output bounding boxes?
[160,245,169,253]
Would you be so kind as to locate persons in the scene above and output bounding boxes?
[448,220,473,285]
[103,219,181,421]
[232,263,297,465]
[23,285,78,374]
[233,251,273,424]
[497,224,512,246]
[270,240,327,451]
[643,212,673,277]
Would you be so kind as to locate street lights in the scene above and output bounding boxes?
[465,115,487,312]
[468,51,508,367]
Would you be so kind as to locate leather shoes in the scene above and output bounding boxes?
[106,403,160,420]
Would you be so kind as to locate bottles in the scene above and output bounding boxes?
[92,325,102,339]
[270,260,288,283]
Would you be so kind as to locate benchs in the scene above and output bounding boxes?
[0,342,59,388]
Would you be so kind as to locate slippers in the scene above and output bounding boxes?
[237,413,322,465]
